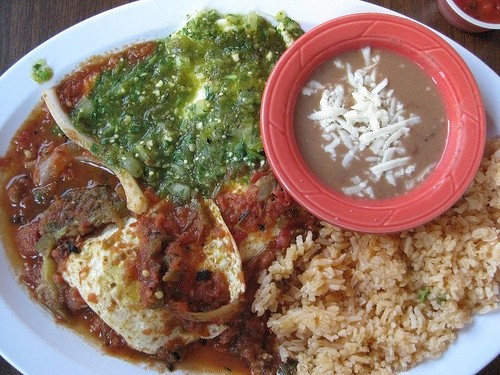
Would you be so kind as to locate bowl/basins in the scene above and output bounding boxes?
[258,13,485,234]
[436,0,500,34]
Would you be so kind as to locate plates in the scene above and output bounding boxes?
[0,0,500,375]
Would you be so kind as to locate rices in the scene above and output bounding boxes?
[249,137,500,375]
